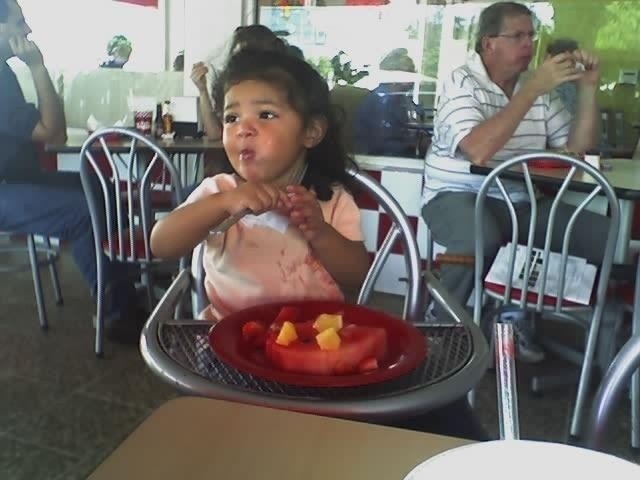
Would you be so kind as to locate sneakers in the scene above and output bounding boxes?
[496,319,546,365]
[89,281,153,341]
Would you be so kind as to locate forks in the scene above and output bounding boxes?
[210,164,309,235]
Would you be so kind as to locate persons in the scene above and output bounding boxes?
[543,38,605,153]
[150,46,370,324]
[0,0,152,342]
[190,24,304,143]
[353,53,422,158]
[420,1,601,365]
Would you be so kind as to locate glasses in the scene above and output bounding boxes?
[484,31,538,43]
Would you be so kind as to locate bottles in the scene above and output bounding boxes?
[162,101,173,137]
[134,111,152,137]
[585,148,600,170]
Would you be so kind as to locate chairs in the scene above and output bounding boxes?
[0,227,63,330]
[80,128,184,356]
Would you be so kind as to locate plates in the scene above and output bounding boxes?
[514,150,579,168]
[207,301,428,386]
[402,440,640,480]
[87,129,134,141]
